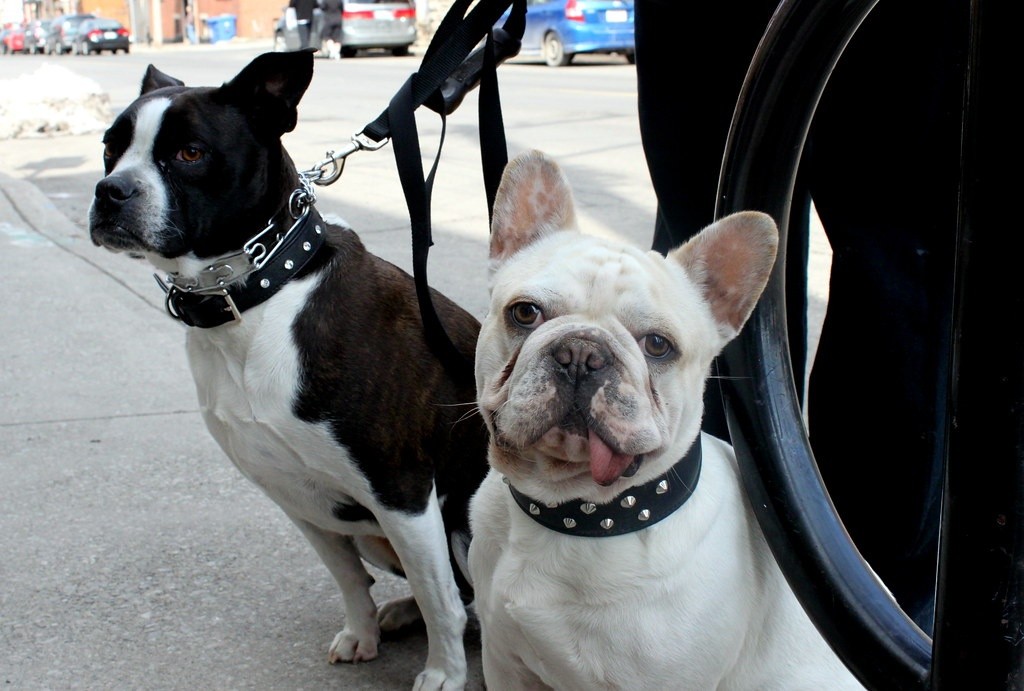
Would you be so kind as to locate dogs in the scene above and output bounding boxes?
[464,147,868,691]
[87,47,483,691]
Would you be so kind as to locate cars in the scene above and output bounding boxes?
[273,0,418,58]
[44,13,95,56]
[491,0,637,67]
[23,20,53,55]
[72,18,130,55]
[3,27,25,55]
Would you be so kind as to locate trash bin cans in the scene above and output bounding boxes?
[206,14,237,44]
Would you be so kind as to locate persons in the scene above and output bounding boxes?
[185,5,195,43]
[289,0,319,48]
[320,0,344,60]
[633,0,810,446]
